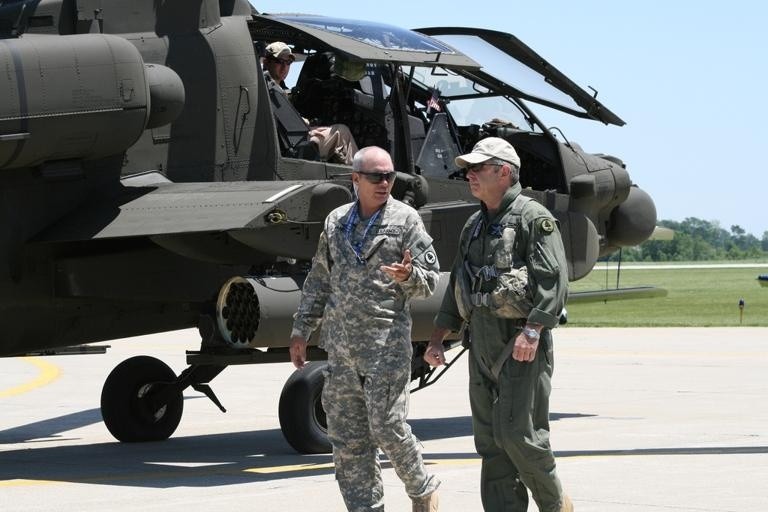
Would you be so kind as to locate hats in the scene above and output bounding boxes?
[264,40,296,62]
[453,135,522,170]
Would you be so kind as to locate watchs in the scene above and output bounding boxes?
[523,328,540,340]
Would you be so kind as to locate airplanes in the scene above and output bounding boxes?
[1,0,658,456]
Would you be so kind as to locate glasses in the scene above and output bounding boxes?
[464,161,505,176]
[355,170,397,186]
[272,57,292,65]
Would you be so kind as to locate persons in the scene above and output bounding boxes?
[422,138,577,511]
[286,146,445,512]
[262,41,359,164]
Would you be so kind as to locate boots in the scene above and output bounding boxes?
[406,484,438,511]
